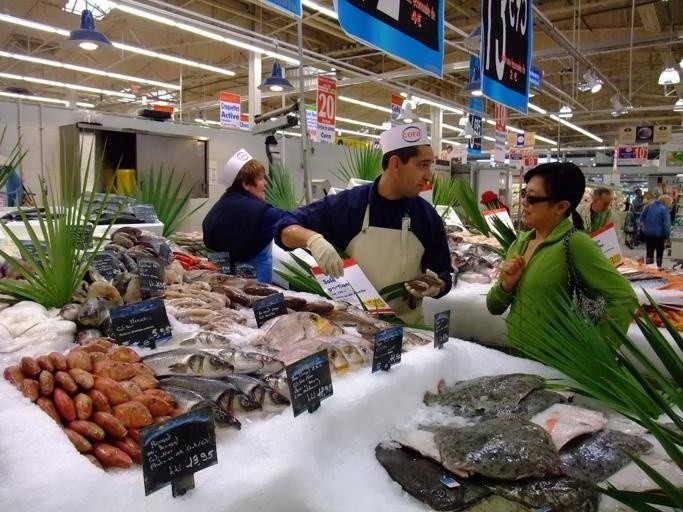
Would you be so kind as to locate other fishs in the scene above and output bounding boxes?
[270,305,378,383]
[59,280,146,349]
[154,327,291,424]
[271,293,380,344]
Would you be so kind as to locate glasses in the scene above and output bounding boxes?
[520,189,550,204]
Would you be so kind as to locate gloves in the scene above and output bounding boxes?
[306,234,344,280]
[404,268,440,298]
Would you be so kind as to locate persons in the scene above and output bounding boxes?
[623,182,674,271]
[485,161,639,365]
[270,120,455,325]
[200,146,290,283]
[567,187,622,243]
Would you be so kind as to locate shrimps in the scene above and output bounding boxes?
[165,279,246,333]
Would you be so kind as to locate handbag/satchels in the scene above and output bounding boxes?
[569,291,605,325]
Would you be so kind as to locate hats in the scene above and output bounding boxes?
[217,149,251,189]
[479,191,497,204]
[379,122,430,155]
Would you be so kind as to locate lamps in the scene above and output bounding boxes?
[60,1,112,55]
[655,6,681,87]
[456,25,481,140]
[257,40,295,97]
[576,63,630,117]
[395,80,419,125]
